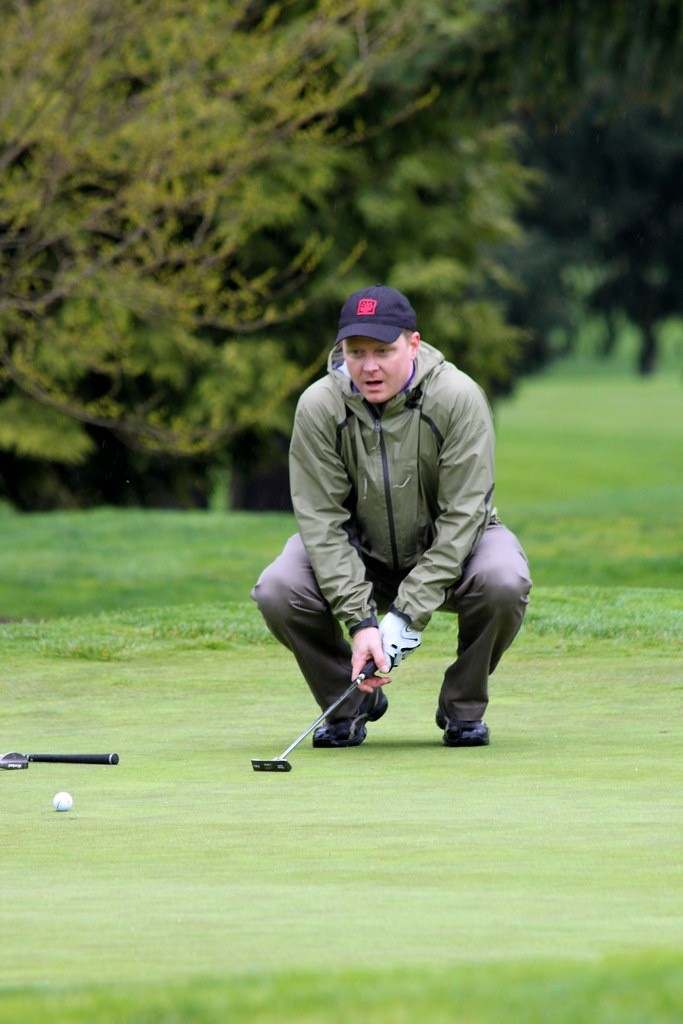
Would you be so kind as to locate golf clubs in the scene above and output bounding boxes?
[1,752,119,770]
[250,658,377,771]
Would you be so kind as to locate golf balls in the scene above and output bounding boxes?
[54,792,72,812]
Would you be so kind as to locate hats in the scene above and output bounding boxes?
[334,284,419,344]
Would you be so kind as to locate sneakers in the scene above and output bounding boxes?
[313,685,388,748]
[436,705,491,747]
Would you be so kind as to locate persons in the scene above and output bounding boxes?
[251,287,531,748]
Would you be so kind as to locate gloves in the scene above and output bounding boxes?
[379,611,421,674]
